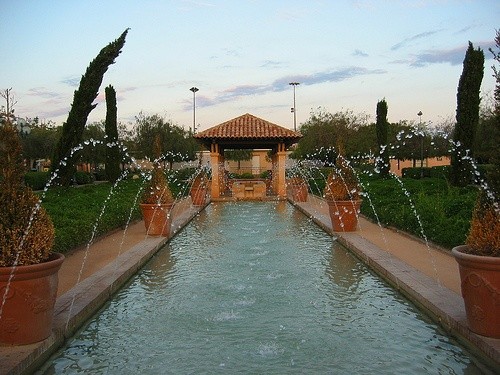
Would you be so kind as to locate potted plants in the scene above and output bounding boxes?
[189,151,209,207]
[291,148,309,202]
[138,135,175,237]
[324,138,364,233]
[0,87,66,348]
[451,152,500,338]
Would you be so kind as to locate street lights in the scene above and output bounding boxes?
[189,87,200,135]
[289,82,300,132]
[417,110,424,178]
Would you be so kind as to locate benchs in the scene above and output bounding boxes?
[232,180,266,197]
